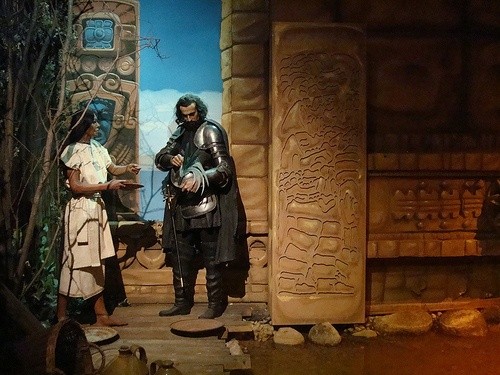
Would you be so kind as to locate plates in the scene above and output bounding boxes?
[168,319,226,334]
[82,326,119,345]
[120,183,145,191]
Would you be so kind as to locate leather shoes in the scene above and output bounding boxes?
[159,304,192,316]
[198,307,228,320]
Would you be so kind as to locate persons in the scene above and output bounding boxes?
[56,109,140,326]
[155,94,248,321]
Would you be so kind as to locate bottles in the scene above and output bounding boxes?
[149,359,183,375]
[103,344,150,375]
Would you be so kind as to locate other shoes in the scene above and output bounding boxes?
[96,315,128,327]
